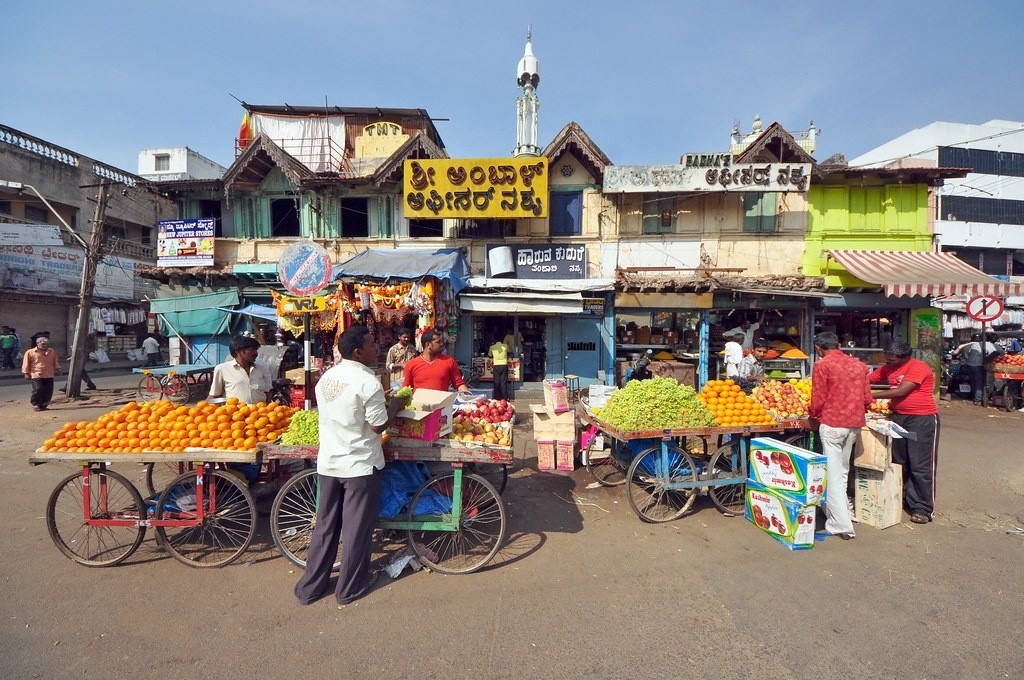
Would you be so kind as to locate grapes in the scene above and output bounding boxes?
[405,420,426,437]
[395,385,418,411]
[281,408,319,446]
[598,376,720,431]
[731,375,754,395]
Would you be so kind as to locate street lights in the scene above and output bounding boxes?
[0,180,94,398]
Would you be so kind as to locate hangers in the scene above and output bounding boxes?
[90,302,146,313]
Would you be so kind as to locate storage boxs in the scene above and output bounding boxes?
[853,427,902,530]
[619,330,699,349]
[745,437,827,549]
[528,378,618,472]
[285,356,323,410]
[385,387,459,442]
[992,395,1005,408]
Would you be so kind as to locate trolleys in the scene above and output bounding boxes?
[580,397,887,524]
[994,373,1024,413]
[29,407,284,569]
[262,417,513,575]
[133,363,217,407]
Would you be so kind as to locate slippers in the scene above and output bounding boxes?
[910,511,930,523]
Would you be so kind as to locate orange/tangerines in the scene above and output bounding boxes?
[698,379,778,426]
[41,400,301,452]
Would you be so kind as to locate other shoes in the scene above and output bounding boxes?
[85,387,96,390]
[32,405,46,412]
[367,568,379,588]
[58,387,66,393]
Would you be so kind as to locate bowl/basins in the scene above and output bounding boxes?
[206,398,227,406]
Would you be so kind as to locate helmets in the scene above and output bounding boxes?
[971,333,983,341]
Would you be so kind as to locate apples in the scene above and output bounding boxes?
[992,354,1024,366]
[442,399,514,445]
[750,378,812,419]
[866,399,892,414]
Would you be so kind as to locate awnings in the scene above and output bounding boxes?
[329,247,472,297]
[826,250,1024,298]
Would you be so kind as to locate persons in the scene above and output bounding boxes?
[806,331,872,540]
[868,341,941,524]
[141,329,163,368]
[720,311,769,381]
[206,336,274,489]
[946,334,1005,406]
[0,326,96,412]
[387,324,524,402]
[294,326,403,606]
